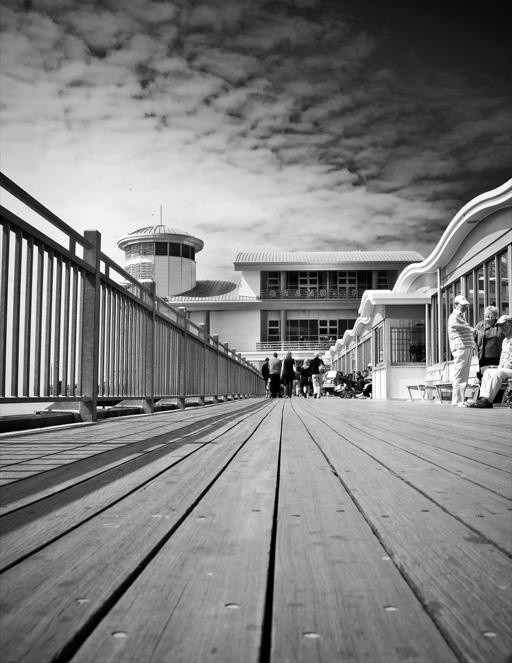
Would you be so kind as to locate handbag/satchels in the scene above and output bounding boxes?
[318,365,327,374]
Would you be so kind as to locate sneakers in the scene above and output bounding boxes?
[452,400,493,408]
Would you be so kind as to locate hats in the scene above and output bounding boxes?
[455,295,470,305]
[494,315,512,327]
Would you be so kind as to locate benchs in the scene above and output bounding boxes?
[405,358,512,408]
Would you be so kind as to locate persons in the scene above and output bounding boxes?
[446,293,483,406]
[467,312,512,409]
[472,303,505,400]
[299,335,305,347]
[260,349,374,400]
[328,336,335,346]
